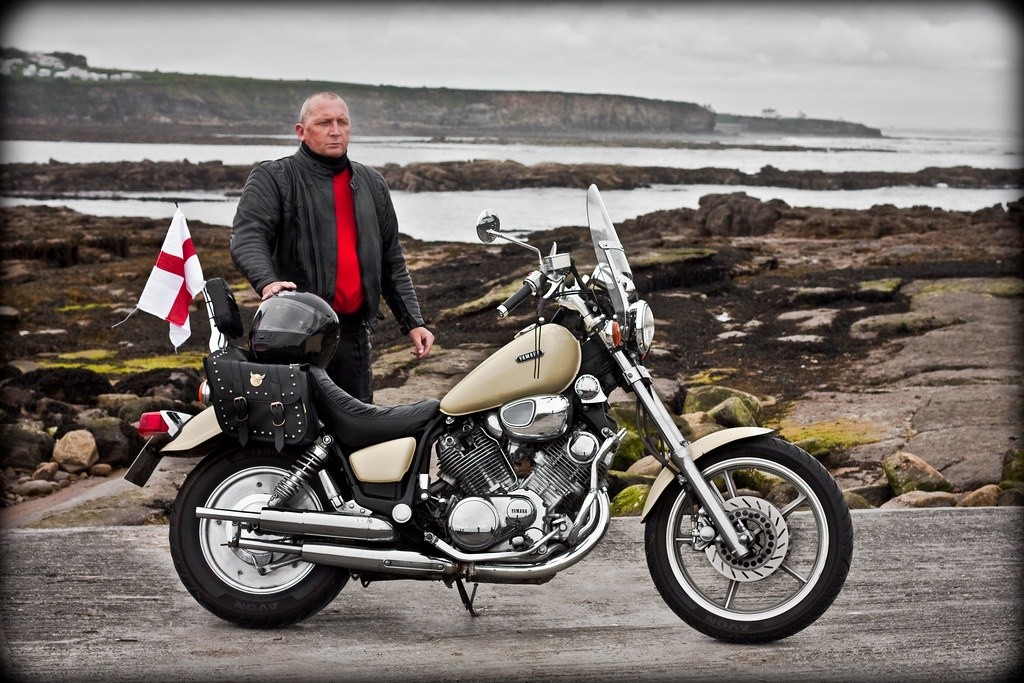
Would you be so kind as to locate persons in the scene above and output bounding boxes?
[230,92,436,405]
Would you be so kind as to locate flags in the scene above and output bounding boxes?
[136,207,207,355]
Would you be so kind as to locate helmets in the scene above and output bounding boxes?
[248,289,340,369]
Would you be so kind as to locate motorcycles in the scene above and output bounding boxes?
[123,183,853,647]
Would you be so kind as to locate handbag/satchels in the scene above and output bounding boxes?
[203,346,320,447]
